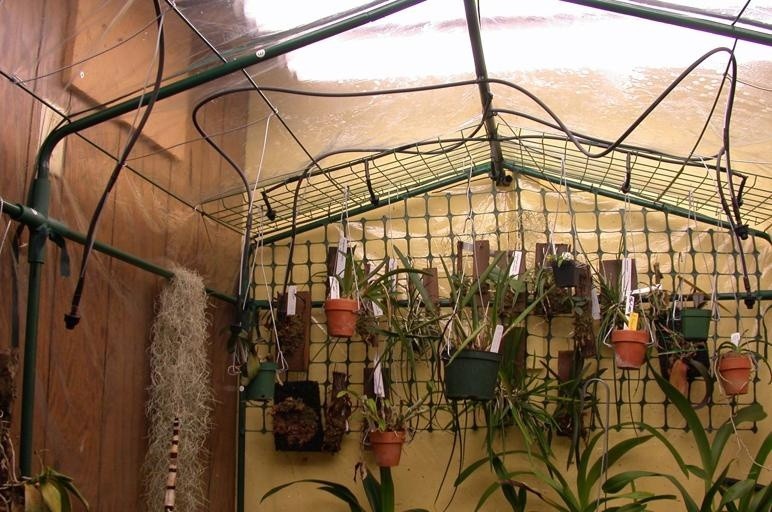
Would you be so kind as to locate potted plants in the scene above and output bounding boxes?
[219,249,595,469]
[595,268,767,396]
